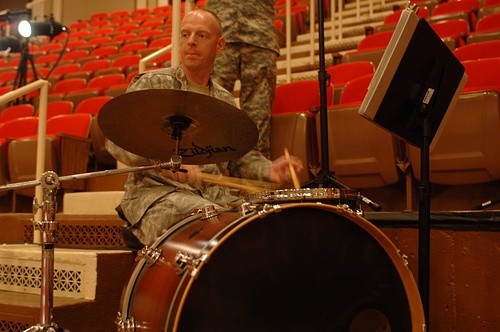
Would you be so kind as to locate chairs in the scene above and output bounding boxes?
[0,0,500,190]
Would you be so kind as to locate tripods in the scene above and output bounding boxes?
[301,0,382,211]
[7,37,38,108]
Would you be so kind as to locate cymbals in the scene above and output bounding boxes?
[97,90,257,167]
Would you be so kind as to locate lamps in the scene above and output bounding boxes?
[17,18,65,39]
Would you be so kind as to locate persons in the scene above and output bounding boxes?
[103,7,303,249]
[206,0,281,160]
[33,13,55,23]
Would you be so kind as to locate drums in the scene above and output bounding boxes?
[119,201,428,332]
[270,187,364,213]
